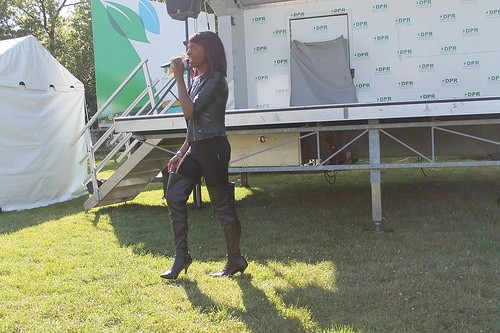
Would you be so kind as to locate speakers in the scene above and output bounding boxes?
[166,0,200,20]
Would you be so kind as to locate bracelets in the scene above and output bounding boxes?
[176,150,184,157]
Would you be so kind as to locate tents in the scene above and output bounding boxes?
[0,34,91,212]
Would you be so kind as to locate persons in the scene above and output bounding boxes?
[157,31,249,279]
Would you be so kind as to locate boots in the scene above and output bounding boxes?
[158,172,196,280]
[204,182,248,277]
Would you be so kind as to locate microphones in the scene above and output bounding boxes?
[161,58,189,68]
